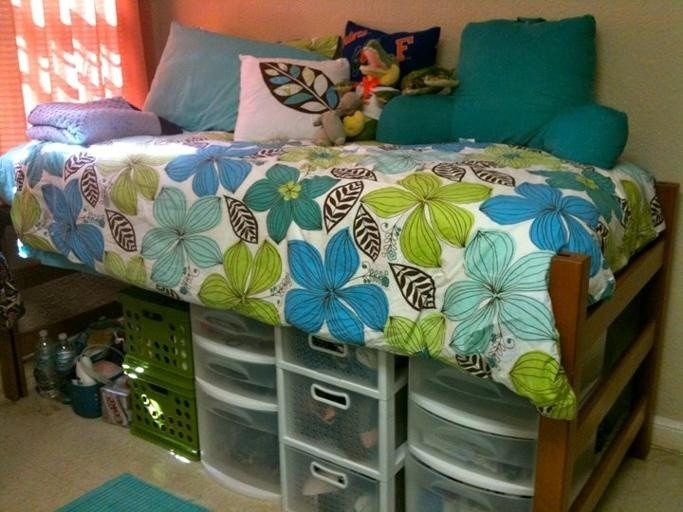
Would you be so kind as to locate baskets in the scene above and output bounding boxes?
[124,369,200,461]
[115,286,194,380]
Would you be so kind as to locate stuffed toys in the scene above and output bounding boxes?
[334,39,400,141]
[307,90,363,147]
[402,63,460,96]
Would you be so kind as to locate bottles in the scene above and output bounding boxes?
[35,329,58,398]
[56,333,77,404]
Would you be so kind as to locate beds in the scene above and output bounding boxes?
[0,131,680,512]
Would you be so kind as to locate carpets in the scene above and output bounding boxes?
[58,474,208,512]
